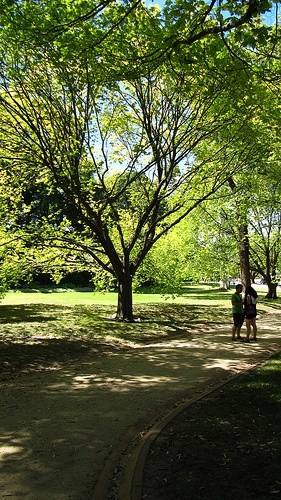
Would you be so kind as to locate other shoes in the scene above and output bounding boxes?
[249,338,257,341]
[236,336,244,339]
[231,338,237,341]
[240,339,249,342]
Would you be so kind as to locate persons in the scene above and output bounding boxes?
[240,286,258,343]
[231,284,245,341]
